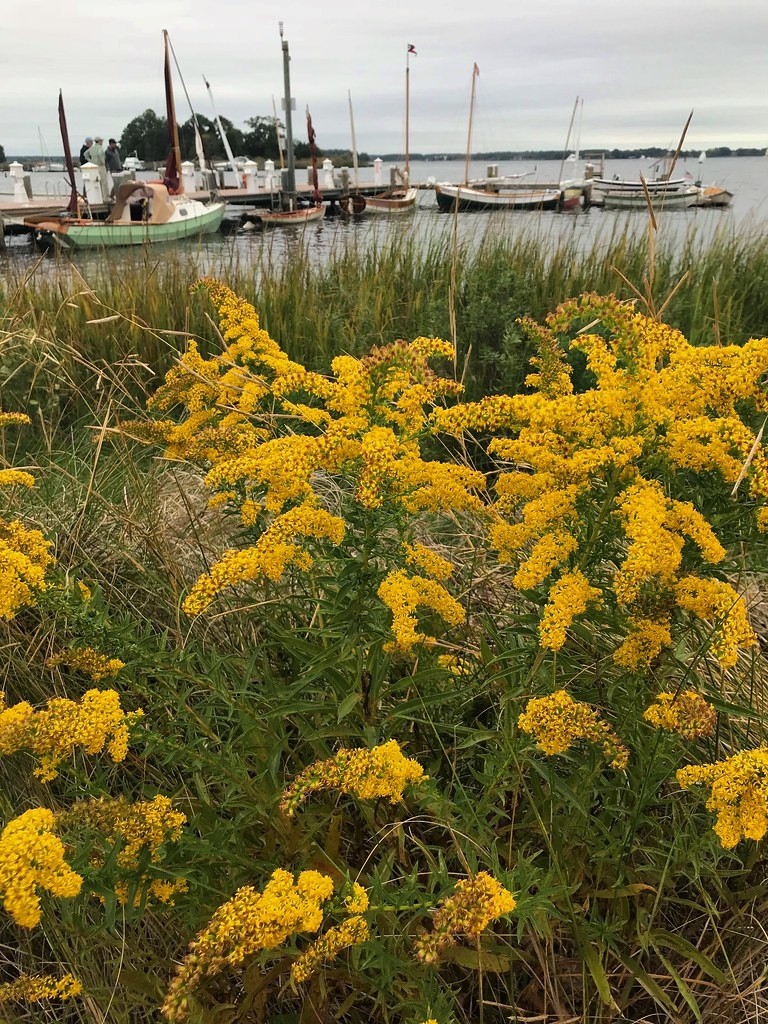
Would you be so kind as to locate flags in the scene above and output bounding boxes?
[474,64,478,74]
[685,172,692,178]
[408,44,417,53]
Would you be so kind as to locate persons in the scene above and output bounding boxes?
[83,136,109,198]
[79,136,93,166]
[104,139,123,200]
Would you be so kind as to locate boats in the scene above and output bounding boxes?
[219,202,258,222]
[692,180,733,206]
[124,149,146,171]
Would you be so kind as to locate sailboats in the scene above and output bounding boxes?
[432,62,586,212]
[337,44,419,214]
[33,127,68,173]
[236,26,328,229]
[590,108,698,209]
[21,27,227,249]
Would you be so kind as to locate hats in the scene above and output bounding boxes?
[93,136,103,141]
[86,137,92,141]
[109,139,117,143]
